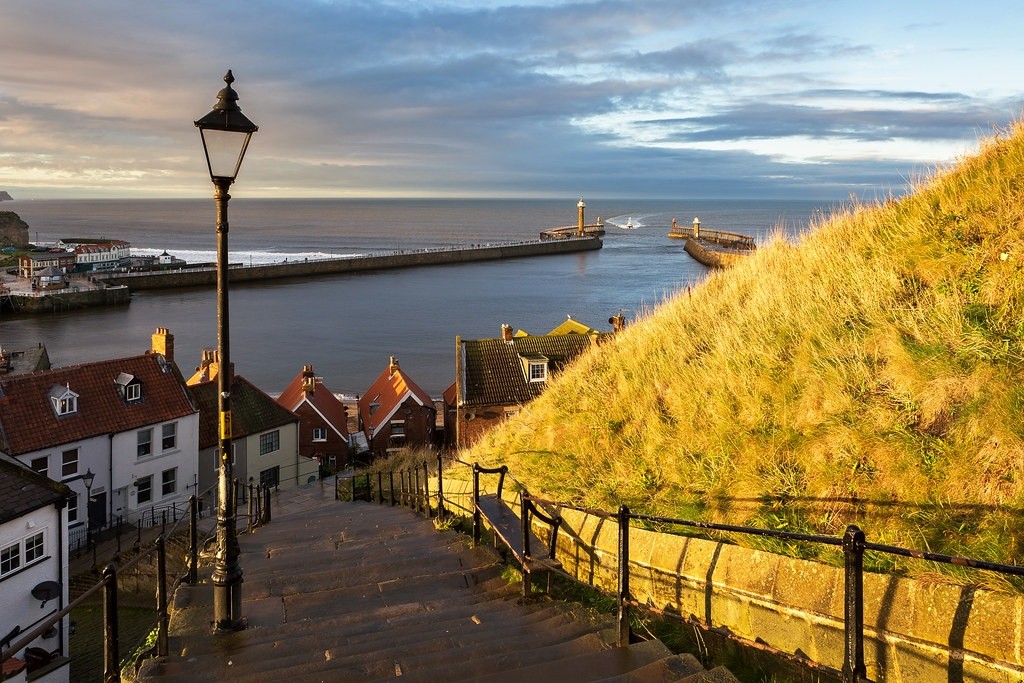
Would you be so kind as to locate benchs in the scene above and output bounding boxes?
[471,464,562,595]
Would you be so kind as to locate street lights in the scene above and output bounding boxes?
[193,69,260,634]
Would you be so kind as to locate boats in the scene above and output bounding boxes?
[627,223,632,228]
